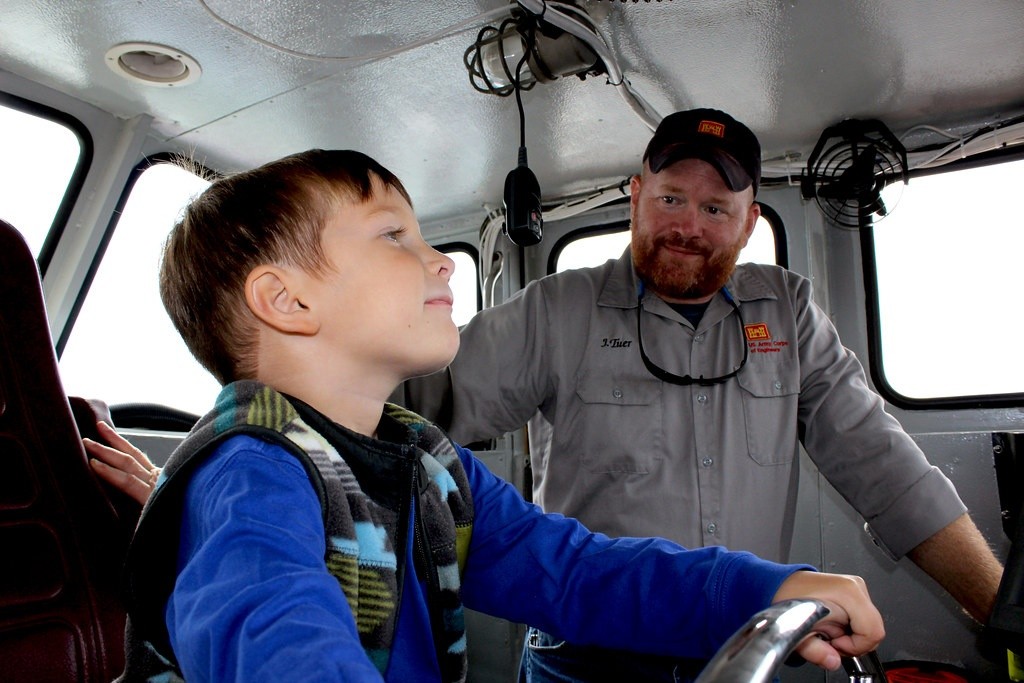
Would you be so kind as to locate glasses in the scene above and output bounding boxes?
[633,279,747,387]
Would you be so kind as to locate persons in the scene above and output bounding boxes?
[113,149,885,683]
[82,109,1006,683]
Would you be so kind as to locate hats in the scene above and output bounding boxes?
[642,108,762,197]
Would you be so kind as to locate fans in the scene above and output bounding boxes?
[799,119,910,234]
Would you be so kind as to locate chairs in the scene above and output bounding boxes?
[0,216,138,683]
